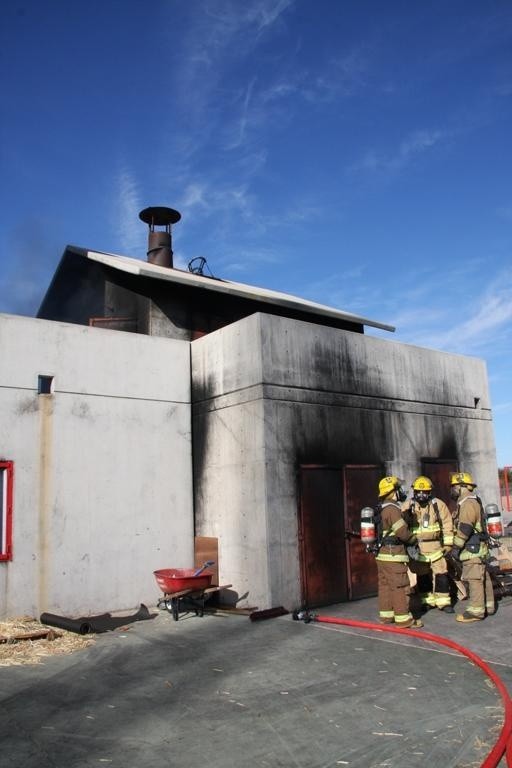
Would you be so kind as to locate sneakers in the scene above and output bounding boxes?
[487,607,495,614]
[410,621,423,627]
[456,612,480,621]
[443,606,455,613]
[421,603,431,610]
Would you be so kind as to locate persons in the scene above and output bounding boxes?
[373,475,423,629]
[448,471,496,622]
[400,474,455,614]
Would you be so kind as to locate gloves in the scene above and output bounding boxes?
[452,545,460,560]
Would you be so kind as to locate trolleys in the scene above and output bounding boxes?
[153,561,233,622]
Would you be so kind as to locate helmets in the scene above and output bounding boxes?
[452,472,477,488]
[413,476,432,490]
[379,476,401,496]
